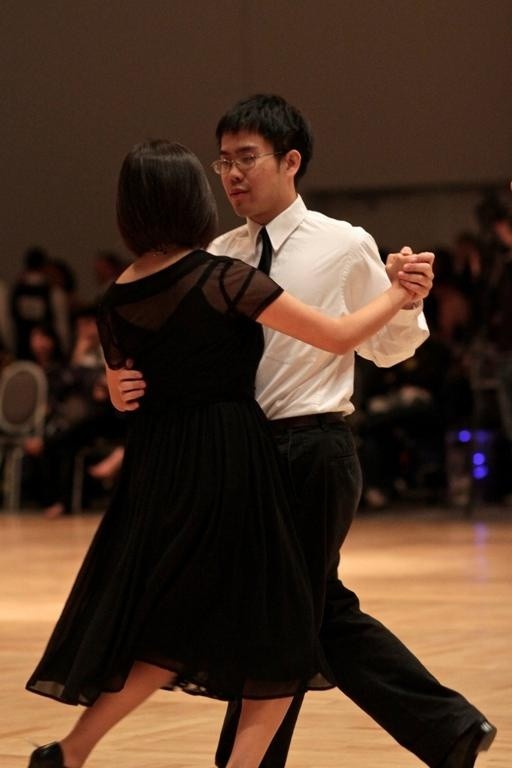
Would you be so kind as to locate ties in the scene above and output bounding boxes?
[256,227,272,279]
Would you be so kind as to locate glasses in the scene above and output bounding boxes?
[210,152,274,175]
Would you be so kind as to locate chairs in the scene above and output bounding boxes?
[0,361,48,510]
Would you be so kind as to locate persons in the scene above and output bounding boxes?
[104,92,497,767]
[1,195,511,525]
[21,142,419,768]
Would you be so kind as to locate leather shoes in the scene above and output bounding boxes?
[28,741,64,768]
[431,716,497,767]
